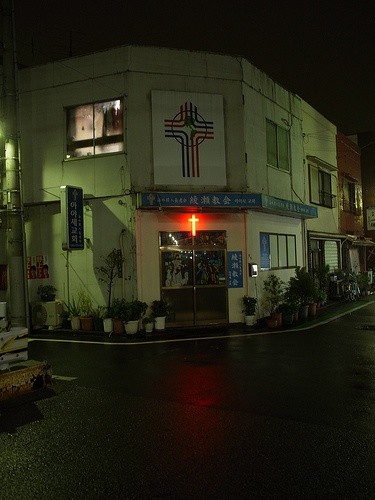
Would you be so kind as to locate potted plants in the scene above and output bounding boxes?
[150,299,169,330]
[279,302,294,326]
[295,265,317,317]
[142,316,156,333]
[263,275,285,327]
[288,277,309,318]
[120,299,145,335]
[240,296,259,326]
[100,306,114,334]
[283,285,300,321]
[93,304,105,331]
[80,296,95,330]
[110,296,129,334]
[37,284,57,302]
[314,267,371,308]
[262,293,279,329]
[62,295,82,331]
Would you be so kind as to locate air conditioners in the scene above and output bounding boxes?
[31,302,64,330]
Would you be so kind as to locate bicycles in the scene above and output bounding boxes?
[343,273,360,302]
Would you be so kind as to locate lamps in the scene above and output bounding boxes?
[188,214,199,237]
